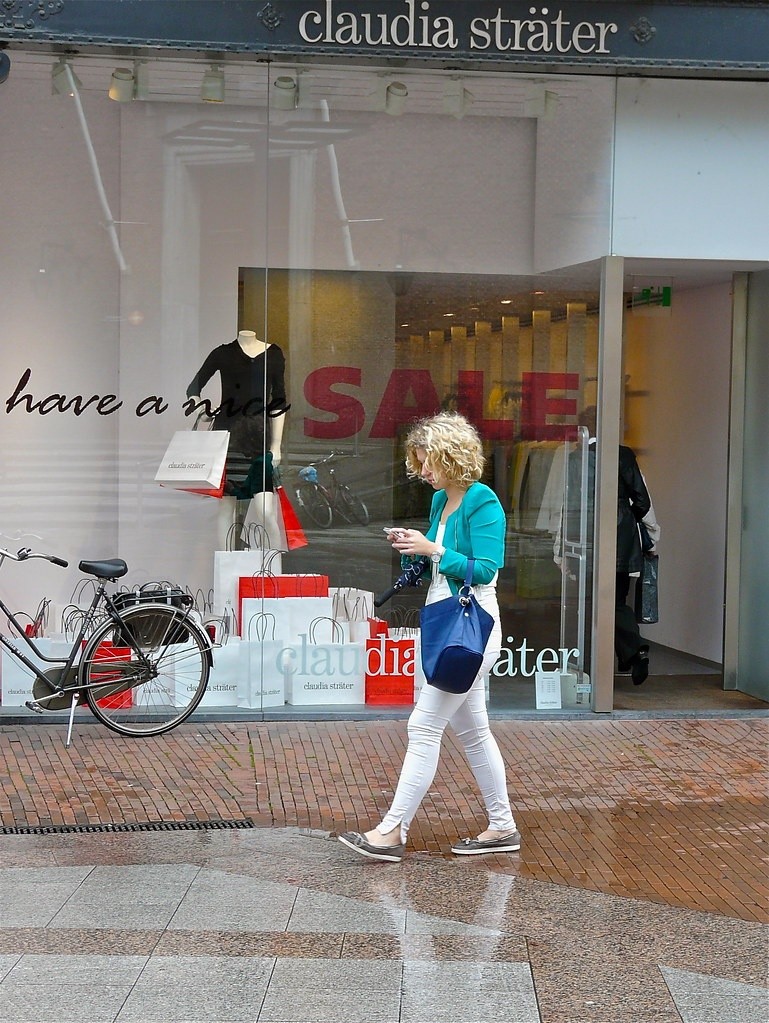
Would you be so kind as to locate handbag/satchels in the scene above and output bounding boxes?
[1,467,414,709]
[633,552,659,624]
[154,407,231,500]
[420,556,496,693]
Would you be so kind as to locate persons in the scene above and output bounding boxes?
[185,330,286,502]
[346,411,520,861]
[554,407,660,685]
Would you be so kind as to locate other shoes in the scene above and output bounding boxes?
[632,648,650,685]
[339,831,406,861]
[452,829,521,854]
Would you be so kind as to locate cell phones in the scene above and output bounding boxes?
[382,527,402,540]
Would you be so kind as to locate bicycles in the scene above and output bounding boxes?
[295,448,371,529]
[0,548,226,750]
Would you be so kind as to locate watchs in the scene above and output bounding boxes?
[430,546,445,563]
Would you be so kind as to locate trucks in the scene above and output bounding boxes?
[490,441,595,610]
